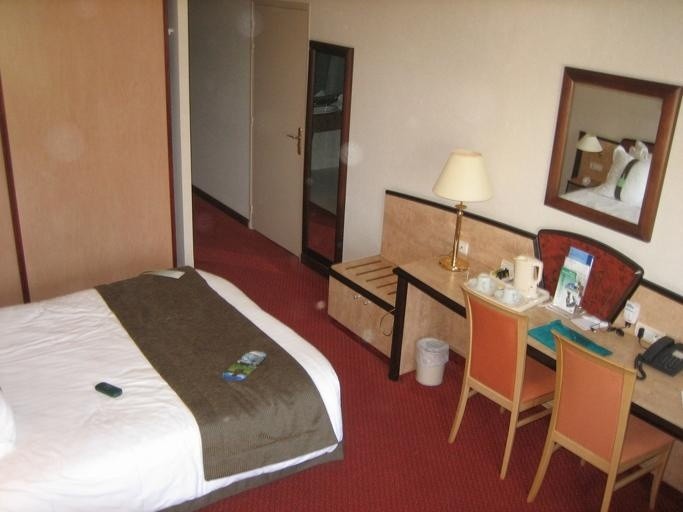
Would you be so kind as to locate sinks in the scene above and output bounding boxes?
[313,105,338,115]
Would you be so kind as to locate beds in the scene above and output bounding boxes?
[563,137,654,226]
[0,263,347,512]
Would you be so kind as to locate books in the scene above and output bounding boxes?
[551,256,594,314]
[568,246,593,269]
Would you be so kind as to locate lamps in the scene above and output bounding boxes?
[573,132,602,155]
[428,145,495,274]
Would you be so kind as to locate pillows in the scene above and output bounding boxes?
[0,387,18,461]
[599,141,651,206]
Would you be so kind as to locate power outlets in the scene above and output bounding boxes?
[632,321,648,341]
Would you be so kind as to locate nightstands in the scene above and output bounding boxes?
[565,175,601,193]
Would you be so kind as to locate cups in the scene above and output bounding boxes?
[501,286,519,304]
[474,273,492,292]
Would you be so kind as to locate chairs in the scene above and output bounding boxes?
[523,326,676,512]
[446,277,560,483]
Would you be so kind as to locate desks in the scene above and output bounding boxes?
[383,250,683,443]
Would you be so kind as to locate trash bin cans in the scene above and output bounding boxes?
[415,337,449,386]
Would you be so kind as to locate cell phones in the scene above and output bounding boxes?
[95,382,122,398]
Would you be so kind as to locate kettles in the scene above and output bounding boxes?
[513,256,544,295]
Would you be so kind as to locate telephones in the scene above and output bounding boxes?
[642,336,683,377]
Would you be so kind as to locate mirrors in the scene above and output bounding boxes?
[300,37,354,274]
[543,64,683,244]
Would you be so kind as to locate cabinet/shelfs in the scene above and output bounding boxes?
[0,1,182,309]
[323,253,402,363]
